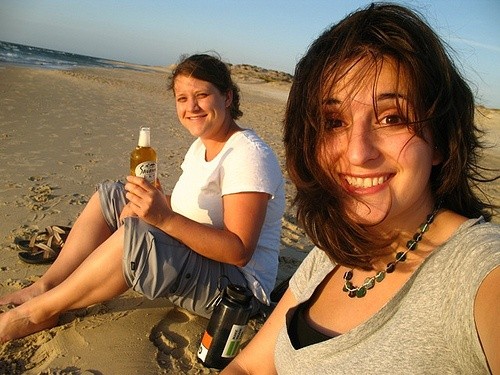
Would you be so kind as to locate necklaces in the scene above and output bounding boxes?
[343,197,442,298]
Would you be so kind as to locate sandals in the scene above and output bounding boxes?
[16,225,72,264]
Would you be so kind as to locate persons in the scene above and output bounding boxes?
[0,51,285,345]
[217,1,500,375]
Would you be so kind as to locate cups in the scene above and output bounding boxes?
[195,285,253,368]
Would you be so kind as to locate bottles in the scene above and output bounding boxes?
[129,126,157,191]
